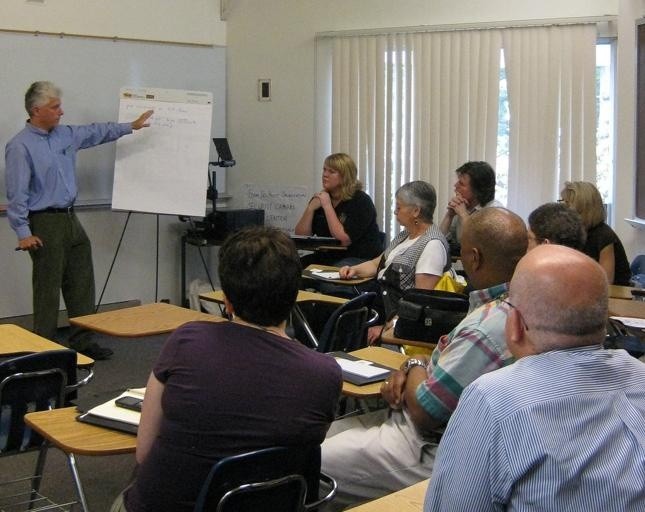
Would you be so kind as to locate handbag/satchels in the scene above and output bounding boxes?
[393,286,470,345]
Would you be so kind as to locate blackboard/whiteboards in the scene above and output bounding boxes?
[0,29,228,215]
[111,87,213,217]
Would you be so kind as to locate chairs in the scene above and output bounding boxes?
[0,233,645,512]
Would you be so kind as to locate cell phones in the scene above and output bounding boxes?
[115,396,143,412]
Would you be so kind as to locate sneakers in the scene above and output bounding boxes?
[84,346,114,360]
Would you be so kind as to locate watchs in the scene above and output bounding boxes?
[399,359,426,372]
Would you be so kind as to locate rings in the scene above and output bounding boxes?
[383,379,390,385]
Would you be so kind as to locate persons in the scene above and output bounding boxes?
[521,205,588,249]
[108,224,343,511]
[2,81,156,363]
[437,158,511,260]
[421,242,644,511]
[317,210,525,511]
[340,181,449,346]
[292,152,386,348]
[557,179,635,287]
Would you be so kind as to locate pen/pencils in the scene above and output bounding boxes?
[15,247,21,251]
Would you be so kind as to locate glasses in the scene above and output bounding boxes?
[499,290,530,333]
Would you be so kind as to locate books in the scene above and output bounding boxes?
[312,270,358,280]
